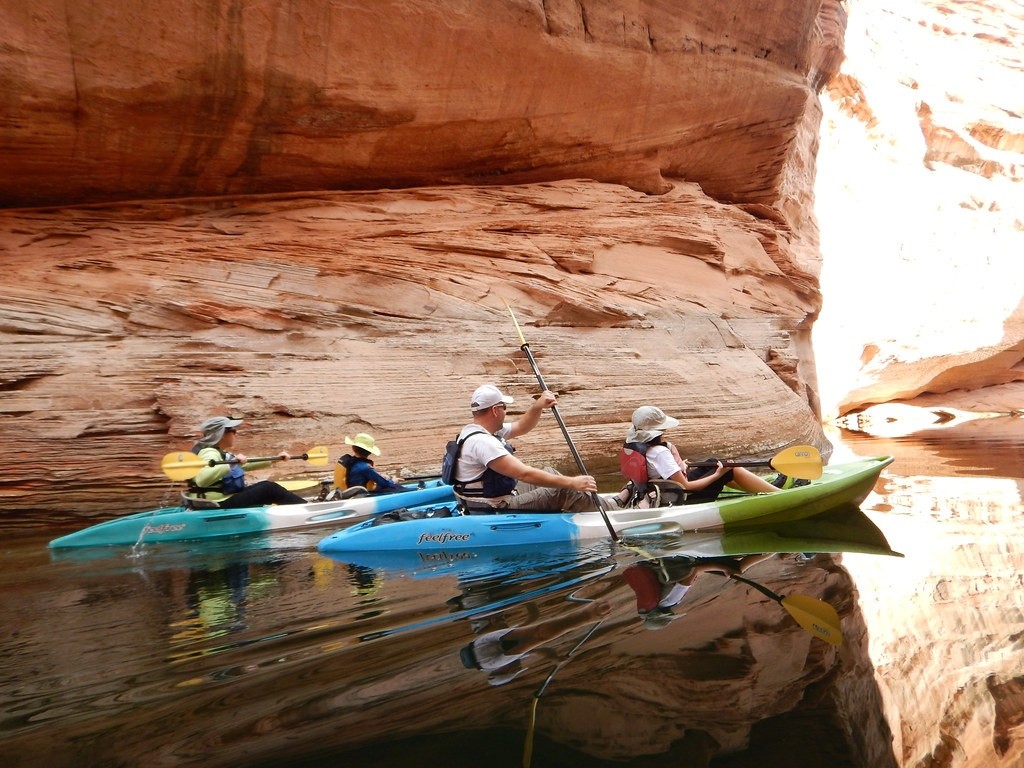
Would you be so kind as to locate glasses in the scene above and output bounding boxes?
[225,429,237,434]
[493,402,507,411]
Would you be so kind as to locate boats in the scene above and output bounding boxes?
[46,473,455,551]
[318,454,895,551]
[322,507,904,571]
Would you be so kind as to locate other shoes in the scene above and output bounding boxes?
[328,489,342,501]
[770,473,787,488]
[789,479,812,491]
[313,485,330,503]
[436,481,443,486]
[416,481,426,491]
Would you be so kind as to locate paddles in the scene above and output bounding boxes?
[498,291,622,545]
[274,472,443,493]
[160,445,330,482]
[686,443,825,481]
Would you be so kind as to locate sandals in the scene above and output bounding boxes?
[612,479,636,509]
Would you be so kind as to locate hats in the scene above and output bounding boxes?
[644,613,687,630]
[471,384,514,412]
[487,666,530,685]
[625,406,679,443]
[345,433,381,456]
[199,416,244,447]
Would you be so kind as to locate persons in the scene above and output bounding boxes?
[637,551,778,630]
[470,600,611,686]
[333,432,424,499]
[624,405,785,507]
[451,384,661,515]
[187,417,311,508]
